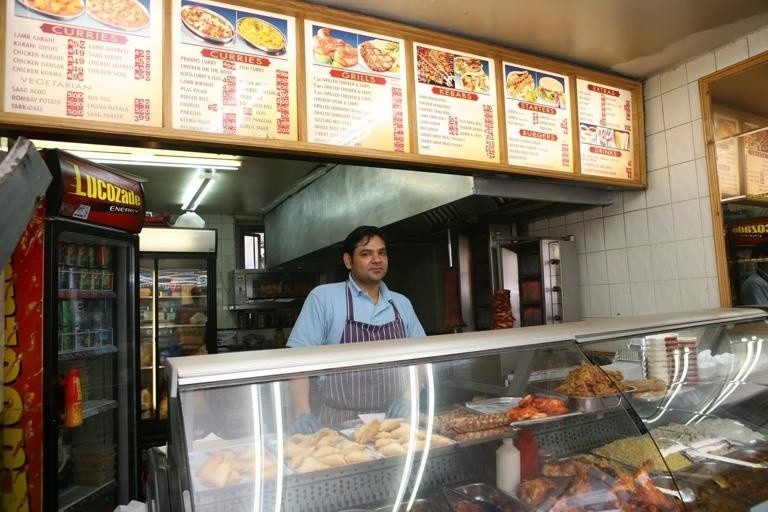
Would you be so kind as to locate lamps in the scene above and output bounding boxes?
[27,139,247,171]
[181,169,218,211]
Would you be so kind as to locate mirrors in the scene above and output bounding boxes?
[697,50,768,308]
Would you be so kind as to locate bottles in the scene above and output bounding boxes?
[488,428,539,490]
[56,241,114,355]
[597,127,614,148]
[238,311,267,329]
[64,368,85,429]
[139,276,205,366]
[615,131,629,150]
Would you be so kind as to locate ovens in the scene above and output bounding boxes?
[229,267,329,304]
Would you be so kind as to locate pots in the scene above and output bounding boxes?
[243,333,265,345]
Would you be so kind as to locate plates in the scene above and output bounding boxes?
[237,18,285,53]
[507,71,535,96]
[87,0,151,32]
[181,4,236,44]
[358,39,401,75]
[18,1,85,22]
[454,57,483,76]
[461,72,490,93]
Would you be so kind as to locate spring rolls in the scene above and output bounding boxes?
[198,417,451,489]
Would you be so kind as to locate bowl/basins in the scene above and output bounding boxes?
[579,125,597,143]
[640,332,734,390]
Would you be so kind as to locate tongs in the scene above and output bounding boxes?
[439,485,502,511]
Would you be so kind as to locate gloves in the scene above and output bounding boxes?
[385,396,410,418]
[288,413,322,436]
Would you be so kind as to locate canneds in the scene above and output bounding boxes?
[57,242,114,290]
[57,299,114,351]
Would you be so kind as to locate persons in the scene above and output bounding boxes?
[285,225,428,436]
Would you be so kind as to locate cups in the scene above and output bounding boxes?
[276,332,284,347]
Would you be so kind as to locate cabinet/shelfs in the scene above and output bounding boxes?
[147,307,768,512]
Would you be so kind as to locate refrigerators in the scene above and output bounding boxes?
[0,152,135,502]
[135,227,215,430]
